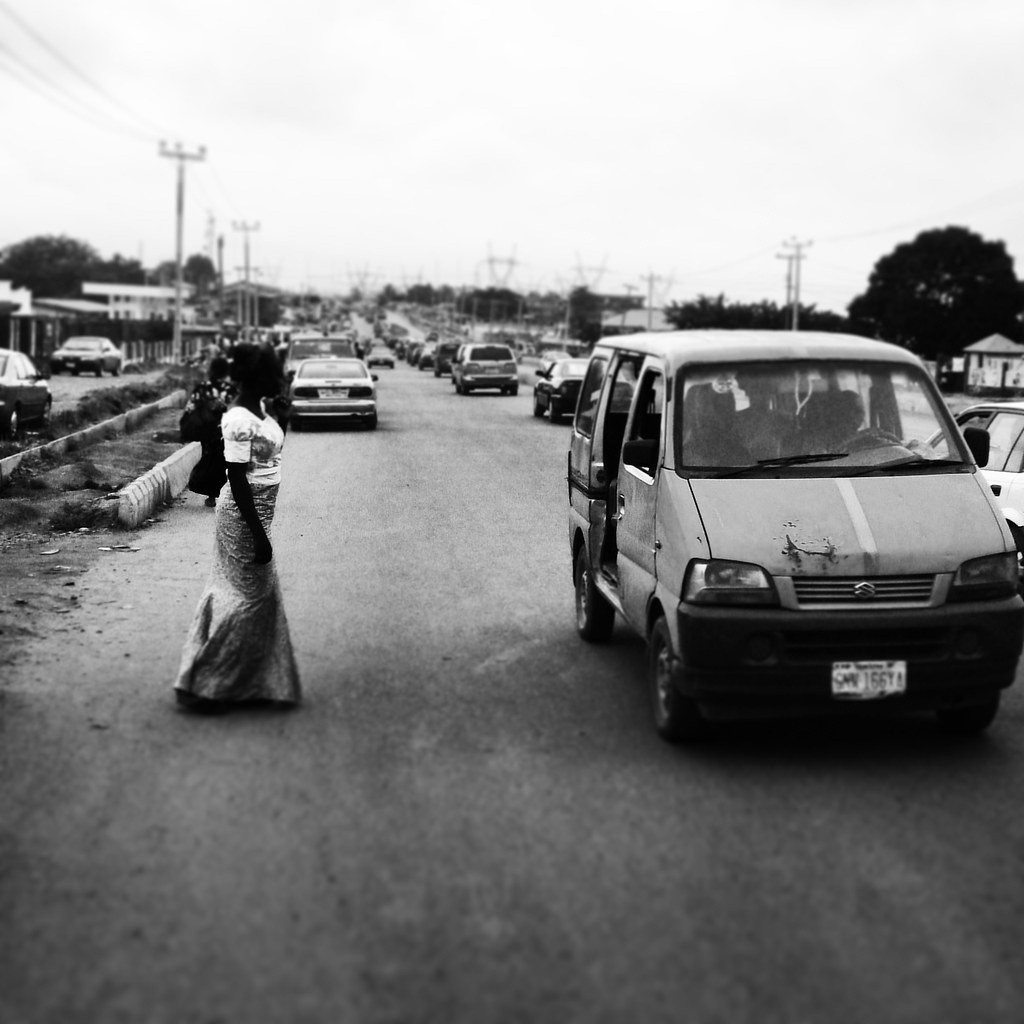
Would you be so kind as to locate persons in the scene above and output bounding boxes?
[733,364,797,462]
[169,342,303,709]
[801,383,874,460]
[182,357,239,501]
[682,390,744,464]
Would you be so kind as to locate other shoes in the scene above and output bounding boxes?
[205,498,216,507]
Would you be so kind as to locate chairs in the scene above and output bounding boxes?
[607,382,860,468]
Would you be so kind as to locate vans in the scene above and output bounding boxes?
[568,329,1023,748]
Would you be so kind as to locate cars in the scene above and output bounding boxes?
[531,358,595,424]
[919,402,1022,569]
[51,335,121,377]
[447,341,518,396]
[0,349,51,444]
[287,360,377,431]
[276,295,463,385]
